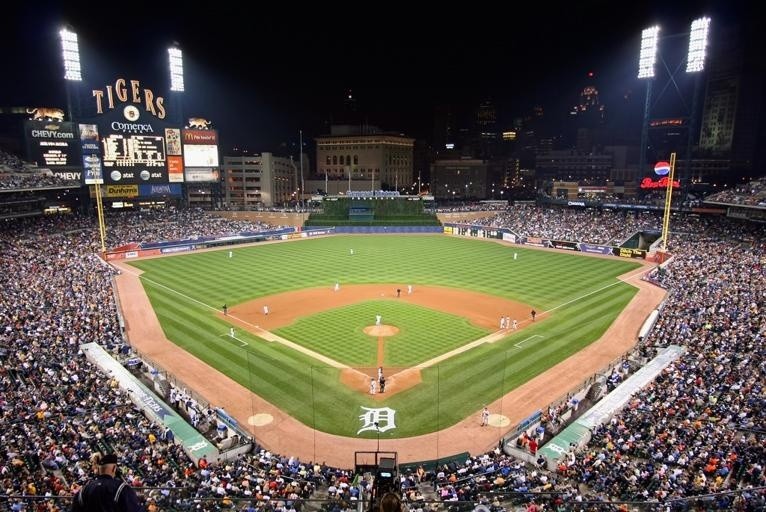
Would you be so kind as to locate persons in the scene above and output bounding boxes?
[1,148,766,510]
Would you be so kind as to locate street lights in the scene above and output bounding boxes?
[58,26,83,142]
[256,190,259,202]
[421,181,504,200]
[637,25,661,178]
[166,47,186,128]
[681,17,711,184]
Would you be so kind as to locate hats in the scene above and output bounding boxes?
[98,454,116,465]
